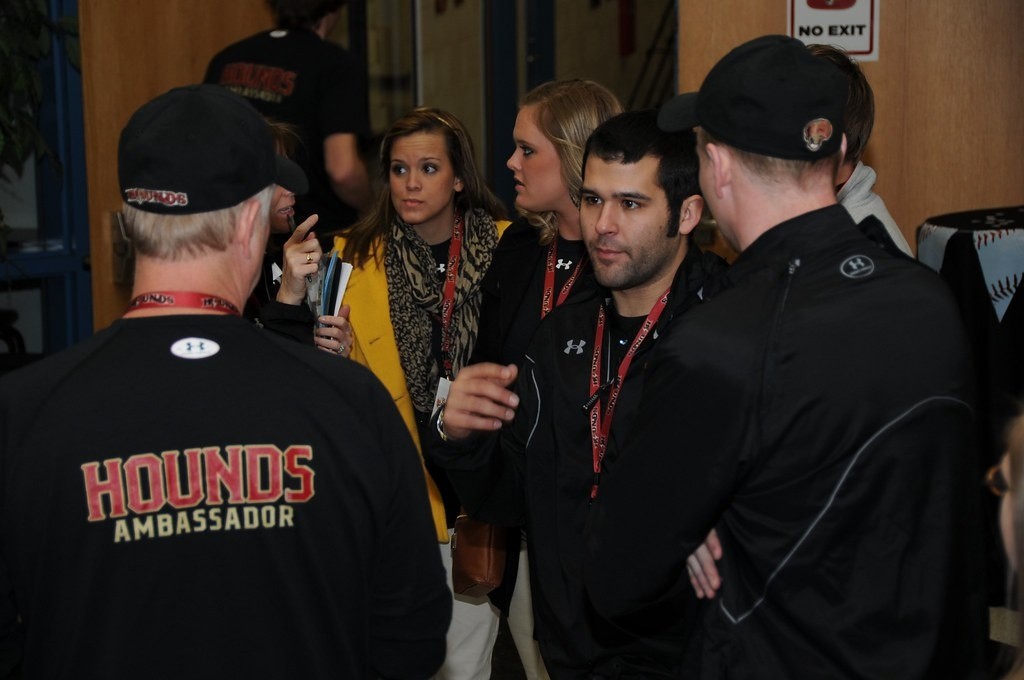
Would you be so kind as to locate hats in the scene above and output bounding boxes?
[118,83,308,215]
[666,33,843,162]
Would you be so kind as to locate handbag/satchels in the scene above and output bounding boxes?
[452,517,504,599]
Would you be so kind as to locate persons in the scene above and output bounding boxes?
[467,75,625,680]
[999,411,1024,680]
[803,41,917,259]
[201,0,371,307]
[422,108,734,680]
[321,106,556,680]
[242,108,354,358]
[0,82,454,680]
[582,32,1023,680]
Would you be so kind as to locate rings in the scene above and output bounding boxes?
[306,253,313,262]
[338,344,345,353]
